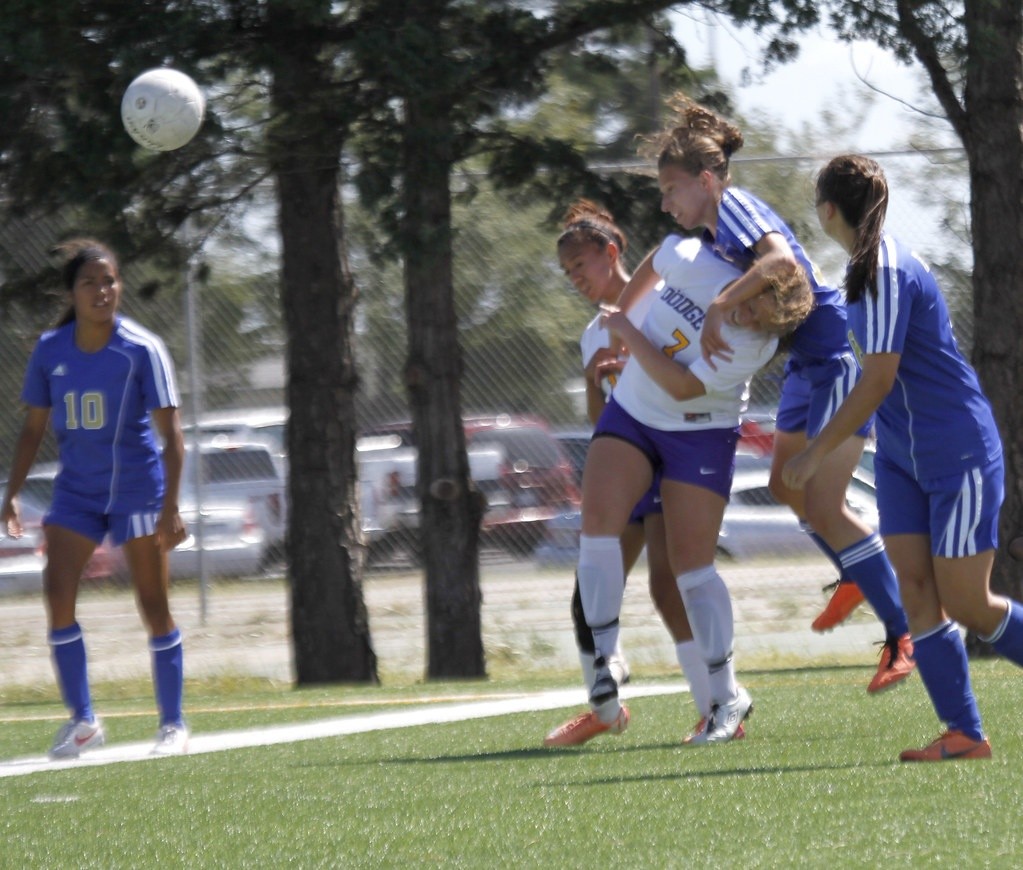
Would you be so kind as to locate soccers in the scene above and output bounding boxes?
[119,67,206,153]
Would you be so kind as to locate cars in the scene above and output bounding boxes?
[1,406,883,603]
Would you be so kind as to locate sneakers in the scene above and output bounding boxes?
[150,725,189,758]
[588,658,631,706]
[542,705,629,747]
[683,716,745,744]
[51,717,103,761]
[898,729,991,764]
[811,575,867,633]
[866,632,917,692]
[689,685,753,745]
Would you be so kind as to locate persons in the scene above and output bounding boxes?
[0,241,188,760]
[552,93,1022,763]
